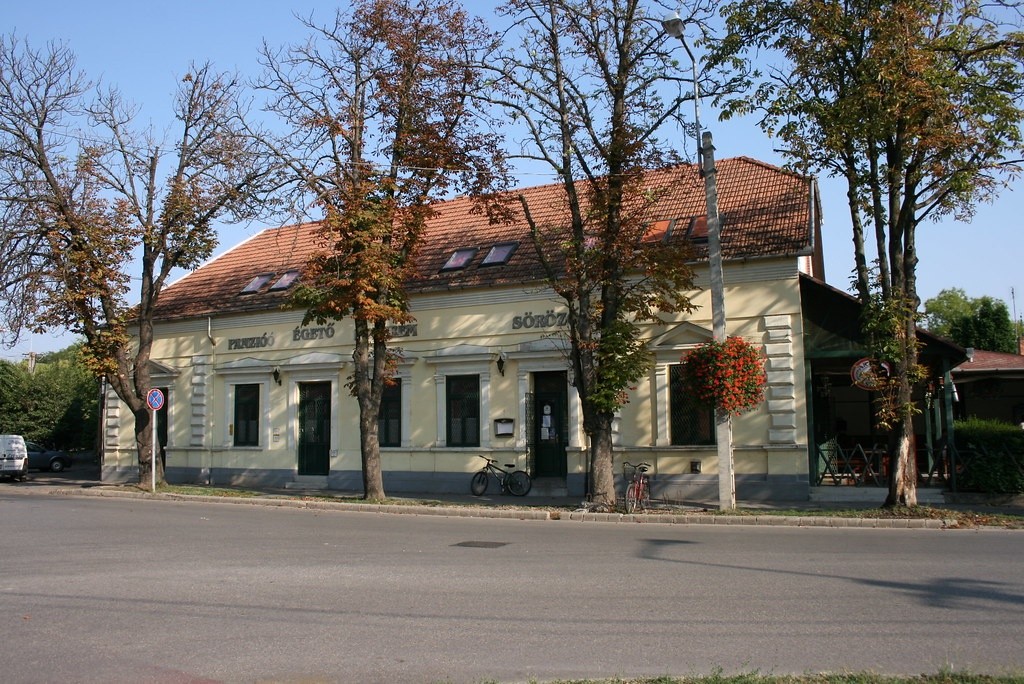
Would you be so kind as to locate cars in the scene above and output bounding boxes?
[25,442,73,473]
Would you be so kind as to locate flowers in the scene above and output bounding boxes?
[679,335,767,422]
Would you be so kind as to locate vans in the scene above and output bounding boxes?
[0,434,30,483]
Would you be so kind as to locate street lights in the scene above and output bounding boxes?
[663,13,737,513]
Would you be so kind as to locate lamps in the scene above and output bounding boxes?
[273,368,282,386]
[690,459,701,474]
[495,356,504,377]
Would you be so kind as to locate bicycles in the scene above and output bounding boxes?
[622,461,652,514]
[470,455,532,498]
